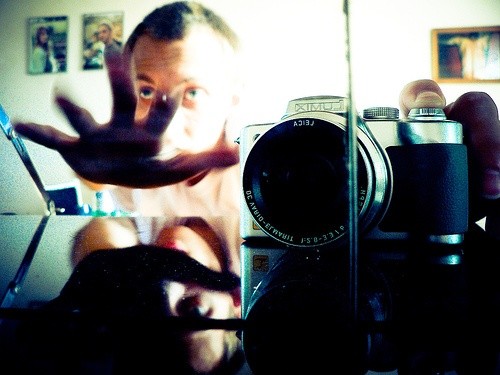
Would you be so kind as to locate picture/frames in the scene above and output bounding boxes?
[431,27,500,83]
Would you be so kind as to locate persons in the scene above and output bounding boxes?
[97,23,123,49]
[68,217,246,375]
[13,0,499,374]
[29,25,60,73]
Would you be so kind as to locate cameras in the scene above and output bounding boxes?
[240,245,461,375]
[239,97,467,248]
